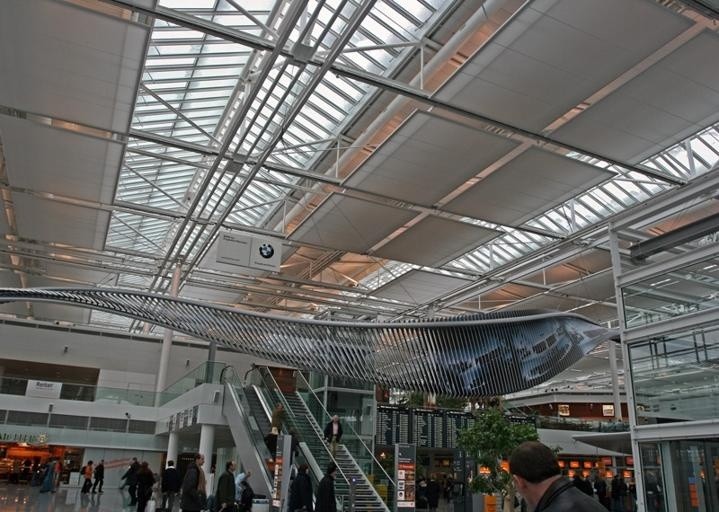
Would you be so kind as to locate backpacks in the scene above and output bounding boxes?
[80,465,88,476]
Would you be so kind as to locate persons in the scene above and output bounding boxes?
[263,427,280,458]
[509,440,608,512]
[573,473,636,512]
[323,413,344,464]
[271,400,285,437]
[315,462,338,511]
[416,475,455,512]
[288,426,300,467]
[287,463,314,511]
[9,451,254,512]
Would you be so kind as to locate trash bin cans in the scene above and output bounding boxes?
[453,496,464,512]
[250,494,269,512]
